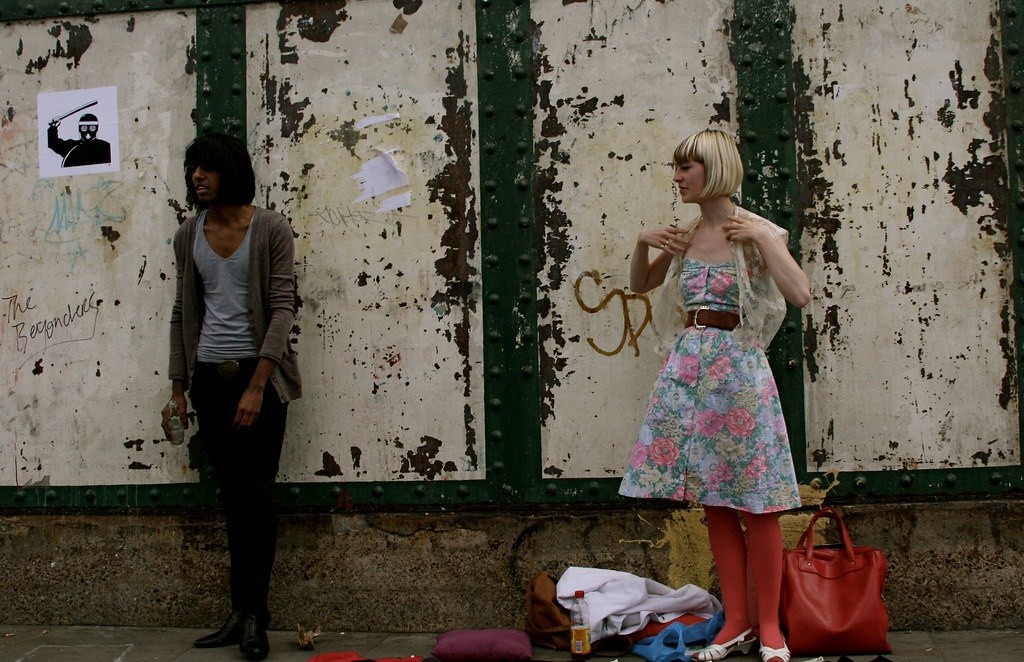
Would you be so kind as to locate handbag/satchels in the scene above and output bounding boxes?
[632,610,727,662]
[525,571,633,657]
[779,507,893,656]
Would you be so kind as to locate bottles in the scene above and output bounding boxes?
[570,590,592,661]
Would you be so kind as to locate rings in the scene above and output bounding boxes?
[664,240,671,246]
[659,243,665,249]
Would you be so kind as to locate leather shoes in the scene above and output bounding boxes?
[194,612,246,648]
[240,616,270,659]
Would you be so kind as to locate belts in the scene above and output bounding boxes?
[193,357,259,379]
[684,307,741,330]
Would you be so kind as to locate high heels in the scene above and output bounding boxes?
[759,626,790,662]
[692,626,758,661]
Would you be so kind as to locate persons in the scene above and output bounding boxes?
[159,131,302,661]
[620,130,811,662]
